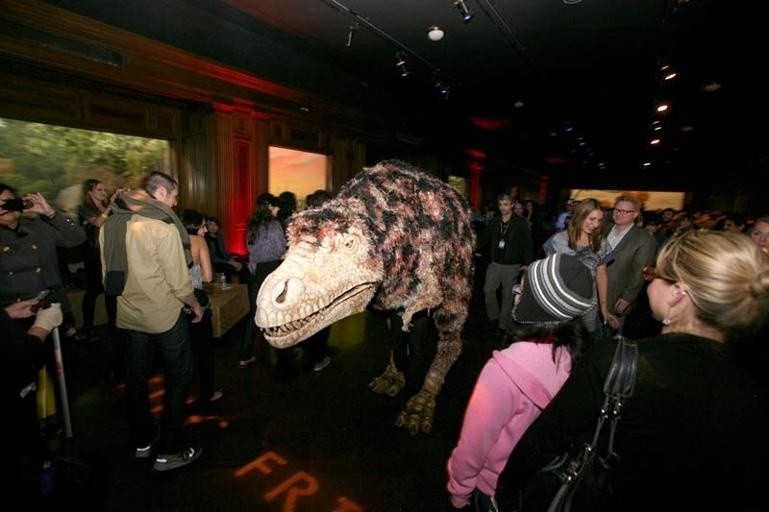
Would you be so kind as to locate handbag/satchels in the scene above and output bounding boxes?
[515,334,640,511]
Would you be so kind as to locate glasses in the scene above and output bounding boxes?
[512,285,521,295]
[642,265,678,289]
[613,208,635,214]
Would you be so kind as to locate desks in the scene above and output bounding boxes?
[202,279,249,338]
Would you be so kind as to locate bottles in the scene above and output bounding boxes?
[220,272,227,288]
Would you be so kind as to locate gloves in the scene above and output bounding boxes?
[29,301,64,334]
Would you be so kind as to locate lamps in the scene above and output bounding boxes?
[426,24,444,41]
[454,0,471,22]
[326,0,451,95]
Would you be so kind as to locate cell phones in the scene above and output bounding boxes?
[33,289,49,300]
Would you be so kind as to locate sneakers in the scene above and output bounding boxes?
[211,391,223,400]
[314,357,332,371]
[241,356,255,366]
[488,319,511,346]
[136,441,205,472]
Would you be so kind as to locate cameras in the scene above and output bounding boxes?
[115,188,127,197]
[1,196,27,213]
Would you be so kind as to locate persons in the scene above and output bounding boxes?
[241,188,334,370]
[1,173,244,475]
[444,195,769,511]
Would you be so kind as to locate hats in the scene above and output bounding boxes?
[512,252,596,324]
